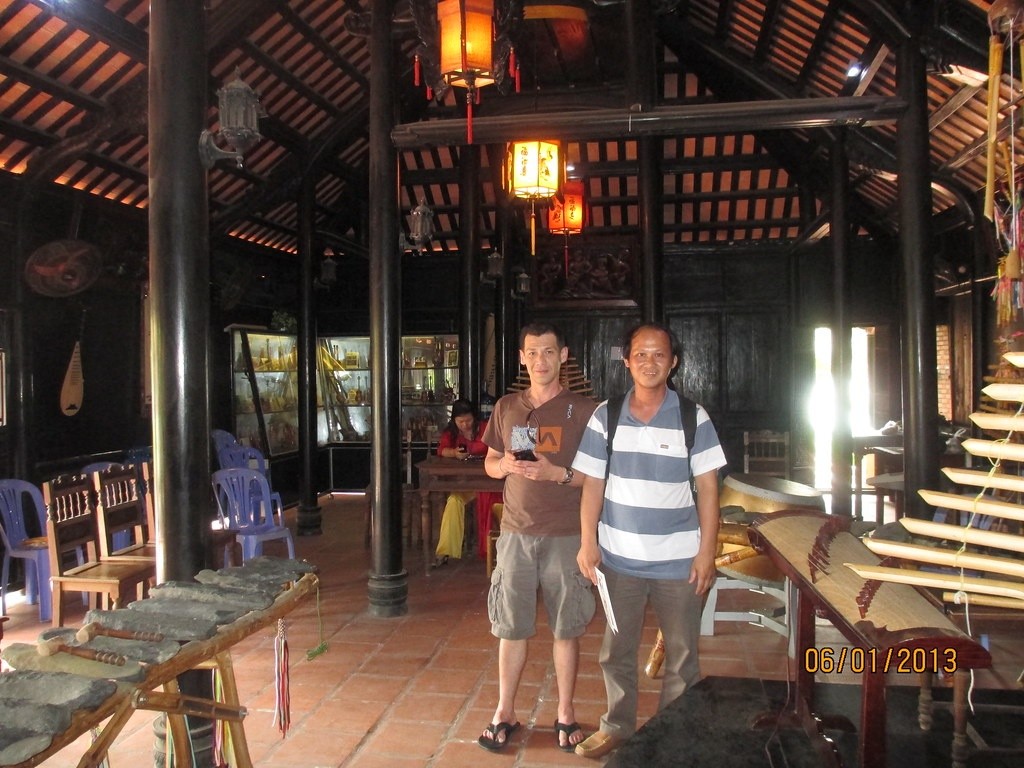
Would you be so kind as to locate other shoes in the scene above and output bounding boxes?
[428,555,448,571]
[574,729,625,757]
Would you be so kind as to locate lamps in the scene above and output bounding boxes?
[196,69,268,172]
[516,271,532,293]
[398,195,439,256]
[486,246,506,279]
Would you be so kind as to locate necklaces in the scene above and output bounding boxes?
[462,432,475,458]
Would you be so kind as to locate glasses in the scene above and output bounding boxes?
[526,408,541,445]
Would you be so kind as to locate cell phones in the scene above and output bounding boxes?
[458,443,467,453]
[513,450,536,462]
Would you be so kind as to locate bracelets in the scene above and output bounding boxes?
[499,456,511,475]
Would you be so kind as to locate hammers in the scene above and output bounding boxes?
[76,621,163,644]
[37,635,125,667]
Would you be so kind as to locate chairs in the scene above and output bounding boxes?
[487,503,506,576]
[741,429,792,479]
[1,429,294,632]
[363,429,414,546]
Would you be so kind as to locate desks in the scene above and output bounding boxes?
[416,457,511,577]
[851,429,904,521]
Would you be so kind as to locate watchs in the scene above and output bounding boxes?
[557,467,574,486]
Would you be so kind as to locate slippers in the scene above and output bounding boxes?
[553,719,585,752]
[477,721,520,750]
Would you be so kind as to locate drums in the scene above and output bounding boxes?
[715,471,826,590]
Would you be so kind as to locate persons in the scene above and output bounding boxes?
[481,322,603,748]
[571,320,727,759]
[432,397,503,568]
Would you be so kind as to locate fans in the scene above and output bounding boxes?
[241,262,278,298]
[22,239,102,297]
